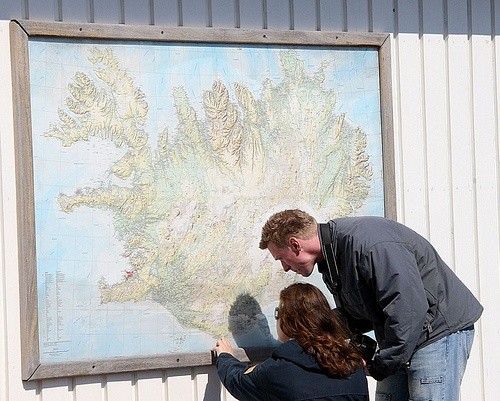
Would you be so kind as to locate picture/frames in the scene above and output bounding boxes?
[8,19,397,382]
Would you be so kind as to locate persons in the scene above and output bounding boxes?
[259,209,484,401]
[210,281,370,401]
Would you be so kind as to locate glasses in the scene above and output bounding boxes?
[274,307,280,320]
